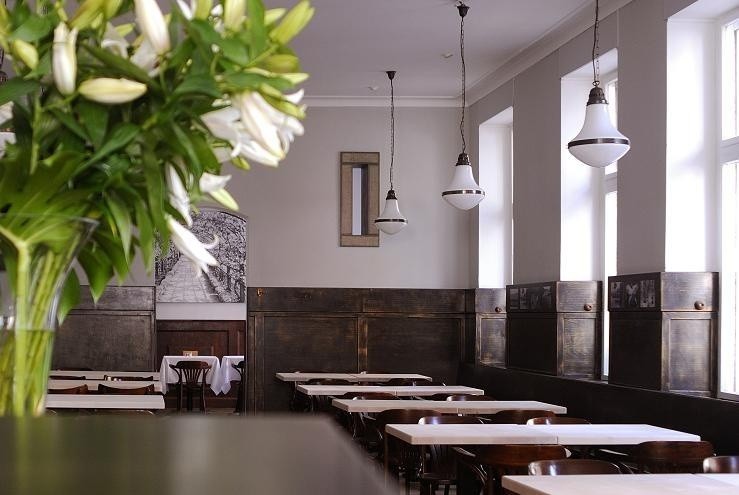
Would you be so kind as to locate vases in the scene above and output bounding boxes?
[0,214,98,419]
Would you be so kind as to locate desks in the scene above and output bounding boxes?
[49,368,160,381]
[220,355,244,413]
[276,373,432,410]
[159,355,221,412]
[0,410,398,495]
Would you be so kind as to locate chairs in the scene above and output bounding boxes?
[169,361,212,416]
[45,376,156,417]
[231,361,244,413]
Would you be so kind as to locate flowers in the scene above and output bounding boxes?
[0,0,315,418]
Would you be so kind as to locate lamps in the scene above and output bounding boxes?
[568,0,631,166]
[373,71,408,235]
[441,0,485,213]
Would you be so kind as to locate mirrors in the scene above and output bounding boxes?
[340,152,380,247]
